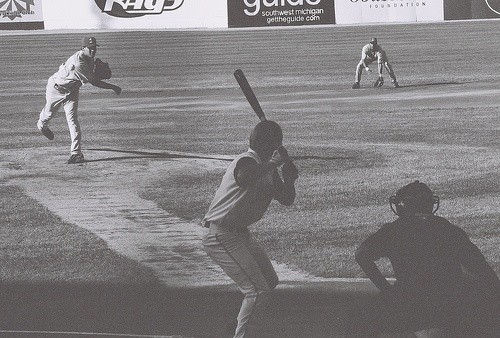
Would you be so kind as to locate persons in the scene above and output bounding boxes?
[355,181,500,338]
[351,37,400,89]
[34,36,122,164]
[201,119,300,338]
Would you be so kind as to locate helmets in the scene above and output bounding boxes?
[389,181,437,217]
[250,120,284,159]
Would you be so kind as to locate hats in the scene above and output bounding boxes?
[369,38,378,45]
[81,35,99,48]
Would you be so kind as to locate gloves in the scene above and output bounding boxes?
[281,160,299,185]
[271,145,291,167]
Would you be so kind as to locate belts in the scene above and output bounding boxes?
[203,220,244,235]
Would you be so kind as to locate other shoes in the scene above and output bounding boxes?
[351,83,360,89]
[67,153,84,164]
[393,81,400,88]
[36,122,55,140]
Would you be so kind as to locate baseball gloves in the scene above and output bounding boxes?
[374,76,384,88]
[95,59,112,79]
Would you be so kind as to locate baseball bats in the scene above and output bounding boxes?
[233,68,298,180]
